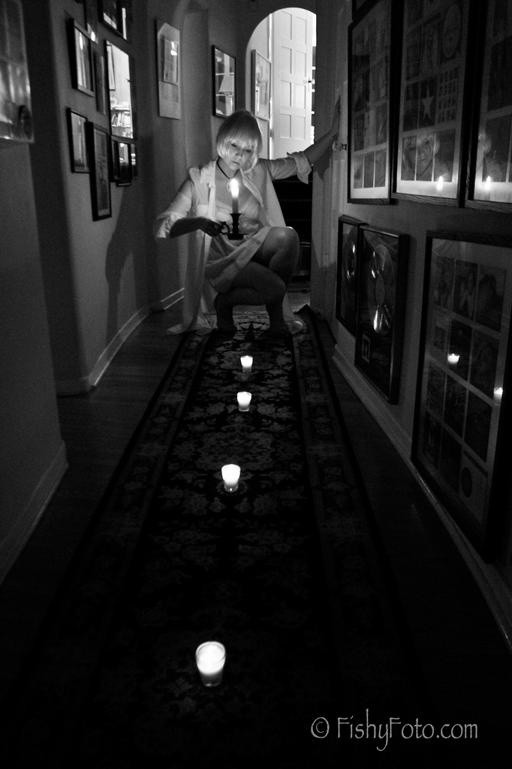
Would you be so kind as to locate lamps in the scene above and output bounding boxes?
[221,464,242,492]
[237,392,252,412]
[195,641,226,687]
[240,355,253,372]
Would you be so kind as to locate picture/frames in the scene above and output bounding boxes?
[334,0,512,573]
[0,0,273,222]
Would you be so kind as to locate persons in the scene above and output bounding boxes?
[151,96,340,336]
[400,129,454,183]
